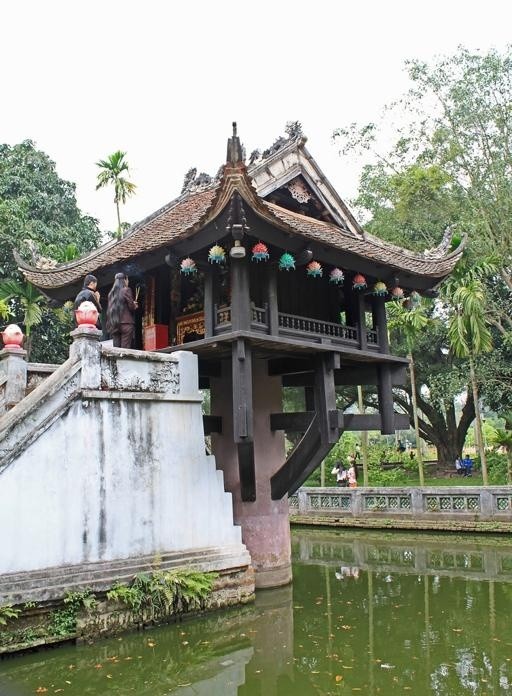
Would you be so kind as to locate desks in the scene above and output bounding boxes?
[175,303,228,345]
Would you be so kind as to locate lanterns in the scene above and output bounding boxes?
[179,243,422,308]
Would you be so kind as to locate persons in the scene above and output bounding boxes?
[330,438,475,487]
[106,272,140,348]
[73,273,102,328]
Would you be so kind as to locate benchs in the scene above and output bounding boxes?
[443,469,470,477]
